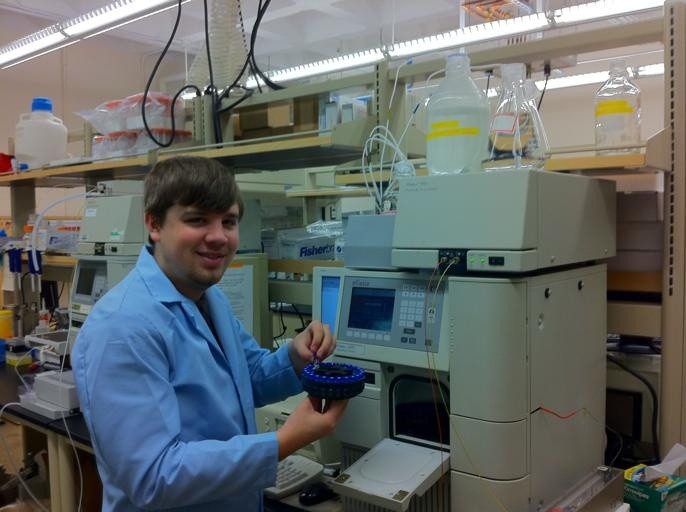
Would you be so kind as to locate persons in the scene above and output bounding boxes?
[69,152,349,512]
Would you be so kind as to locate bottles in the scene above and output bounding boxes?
[51,220,79,249]
[14,96,67,162]
[486,61,553,173]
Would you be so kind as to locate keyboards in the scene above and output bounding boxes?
[263,454,326,500]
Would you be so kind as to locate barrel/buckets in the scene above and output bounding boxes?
[12,95,70,170]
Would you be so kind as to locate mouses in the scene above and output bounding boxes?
[299,481,332,506]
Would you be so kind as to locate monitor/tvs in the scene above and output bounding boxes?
[311,265,347,343]
